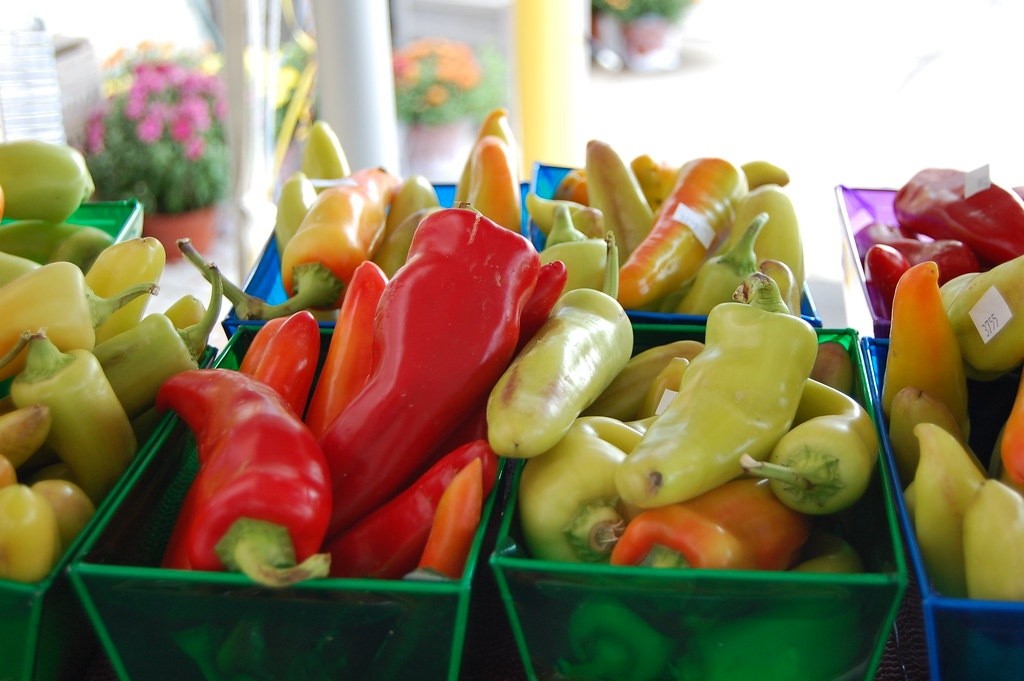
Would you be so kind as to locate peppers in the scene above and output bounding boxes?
[485,141,883,681]
[0,109,567,681]
[864,168,1024,605]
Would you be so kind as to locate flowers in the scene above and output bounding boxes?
[391,34,482,126]
[71,38,227,212]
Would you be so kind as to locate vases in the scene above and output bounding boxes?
[144,202,217,264]
[403,119,474,177]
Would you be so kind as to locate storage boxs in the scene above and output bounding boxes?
[2,164,1024,680]
